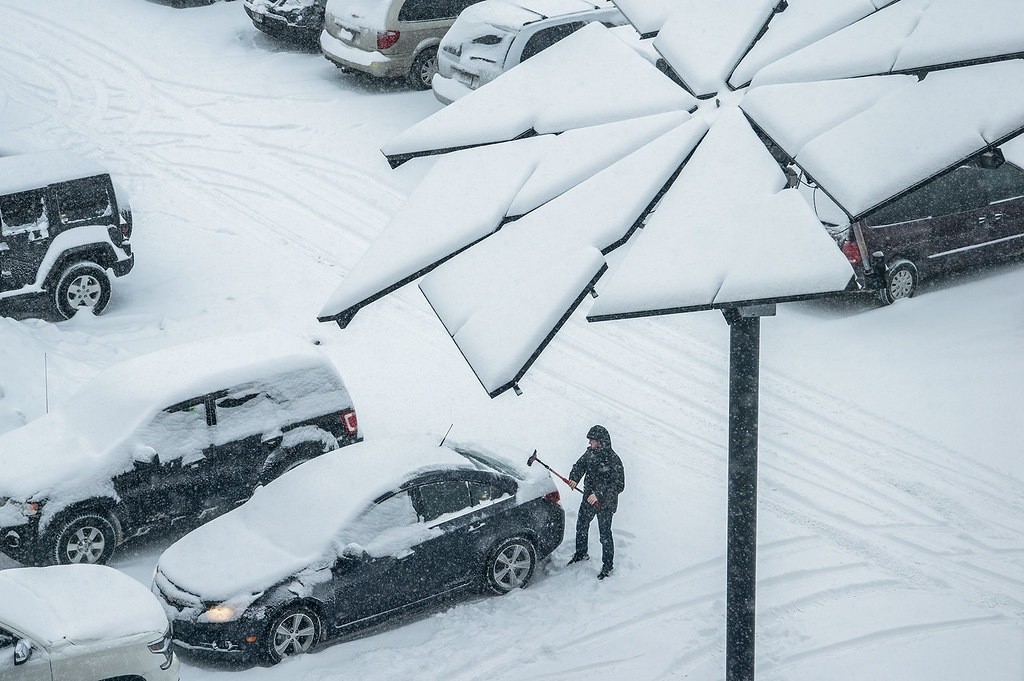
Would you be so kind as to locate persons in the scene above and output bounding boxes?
[566,425,625,580]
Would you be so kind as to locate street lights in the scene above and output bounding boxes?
[315,0,1024,681]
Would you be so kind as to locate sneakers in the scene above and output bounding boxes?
[566,553,589,566]
[597,567,614,580]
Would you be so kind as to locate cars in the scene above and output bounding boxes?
[151,441,567,668]
[0,561,182,681]
[244,0,692,108]
[784,147,1024,306]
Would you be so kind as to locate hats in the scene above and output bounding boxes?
[587,425,611,442]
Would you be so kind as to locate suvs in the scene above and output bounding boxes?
[0,327,366,569]
[0,150,136,322]
